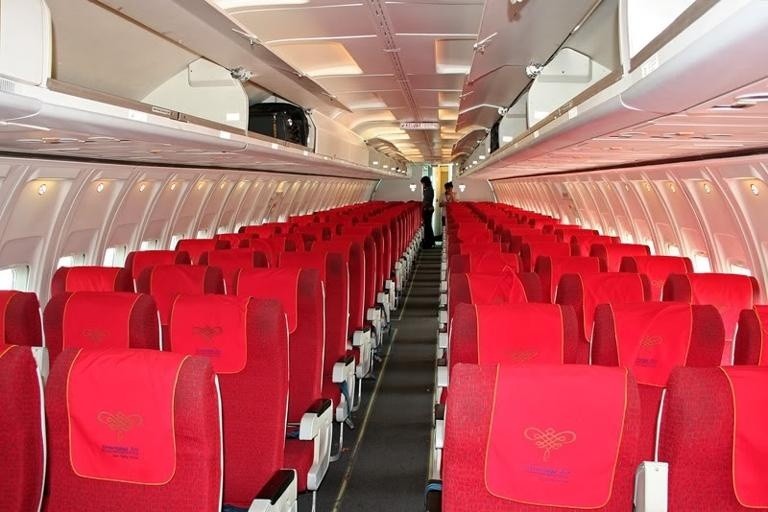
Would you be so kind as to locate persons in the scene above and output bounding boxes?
[439,181,460,231]
[421,176,437,249]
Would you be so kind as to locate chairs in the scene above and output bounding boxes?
[442,199,598,247]
[558,271,645,365]
[136,265,223,351]
[442,254,524,303]
[45,347,224,512]
[525,241,571,271]
[621,256,696,303]
[589,243,651,272]
[232,270,333,512]
[439,274,543,365]
[593,304,725,461]
[161,297,299,512]
[735,305,768,364]
[50,265,130,300]
[654,365,768,512]
[535,256,601,305]
[199,253,266,296]
[436,305,580,419]
[45,293,158,364]
[571,236,620,257]
[428,363,639,511]
[310,242,372,411]
[270,254,346,459]
[175,240,228,265]
[0,288,43,359]
[0,344,44,512]
[663,273,760,366]
[442,241,505,268]
[218,200,426,254]
[125,252,190,292]
